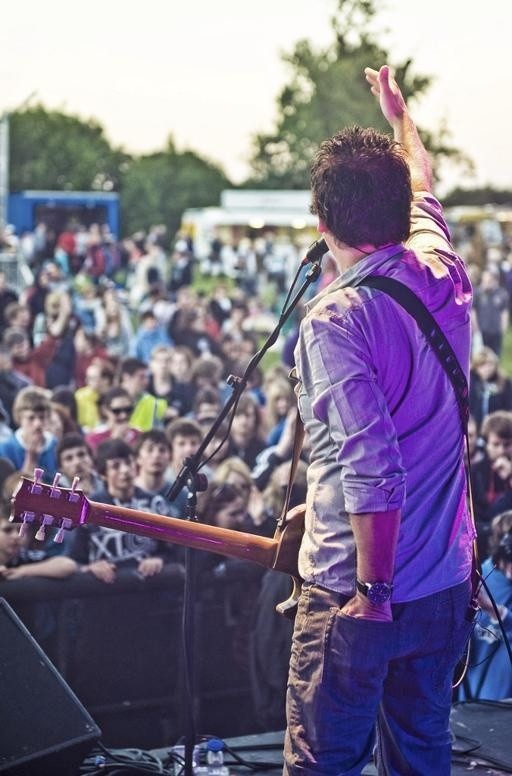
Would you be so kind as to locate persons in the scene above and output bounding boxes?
[280,61,481,775]
[1,218,512,707]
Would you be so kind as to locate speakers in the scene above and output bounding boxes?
[0,596,102,776]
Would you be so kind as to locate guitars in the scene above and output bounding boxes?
[10,468,480,690]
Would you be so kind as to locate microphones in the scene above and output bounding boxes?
[300,235,330,265]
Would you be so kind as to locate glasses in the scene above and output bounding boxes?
[112,407,133,414]
